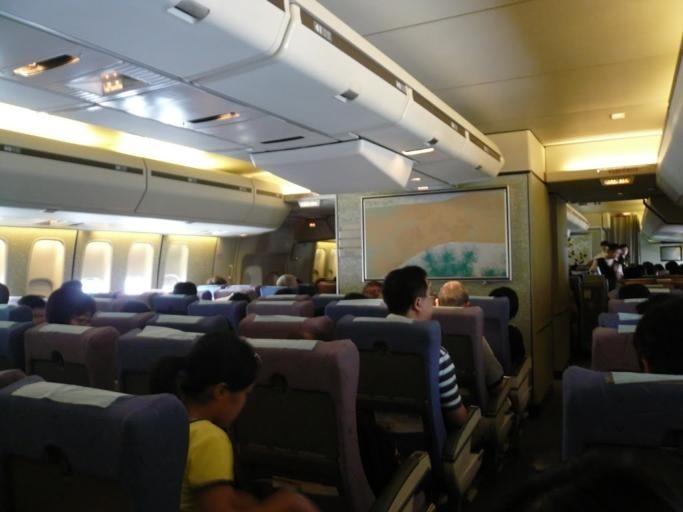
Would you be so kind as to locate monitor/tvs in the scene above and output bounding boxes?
[659,245,683,262]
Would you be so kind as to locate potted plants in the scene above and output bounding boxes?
[567,237,587,271]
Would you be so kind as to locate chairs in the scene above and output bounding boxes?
[564,271,683,510]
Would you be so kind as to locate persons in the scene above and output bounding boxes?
[586,240,682,377]
[0,271,528,503]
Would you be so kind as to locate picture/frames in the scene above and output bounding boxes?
[660,246,682,261]
[360,183,512,283]
